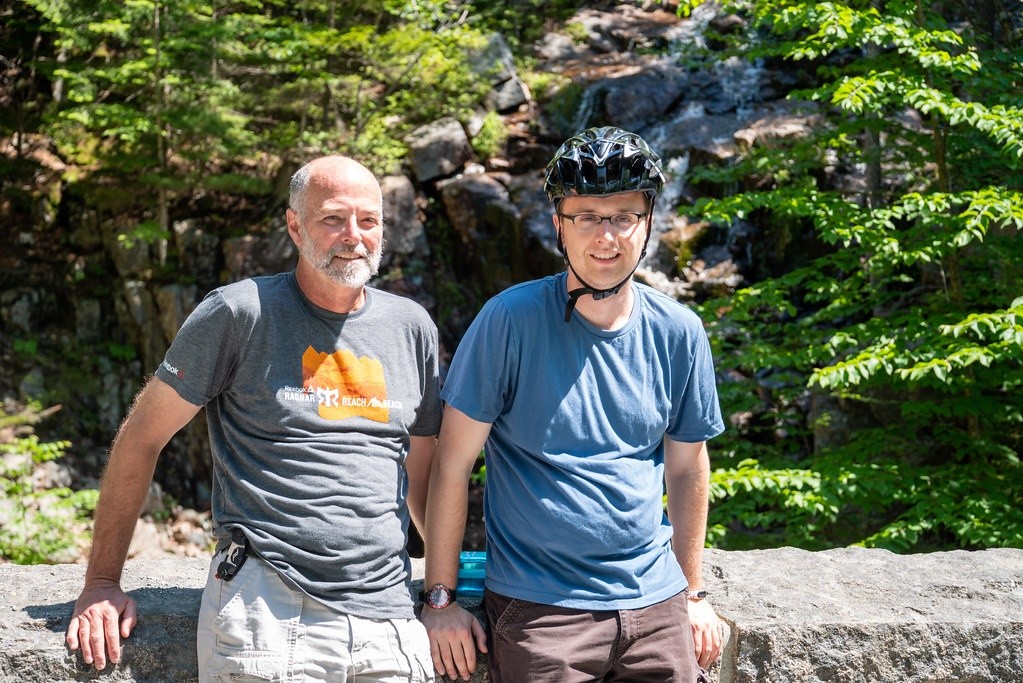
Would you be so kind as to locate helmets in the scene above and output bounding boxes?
[544,125,666,209]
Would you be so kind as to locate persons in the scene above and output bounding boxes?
[420,126,726,683]
[66,154,443,683]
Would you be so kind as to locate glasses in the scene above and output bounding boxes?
[559,212,648,232]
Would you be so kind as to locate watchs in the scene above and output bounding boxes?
[688,591,707,600]
[419,584,457,608]
